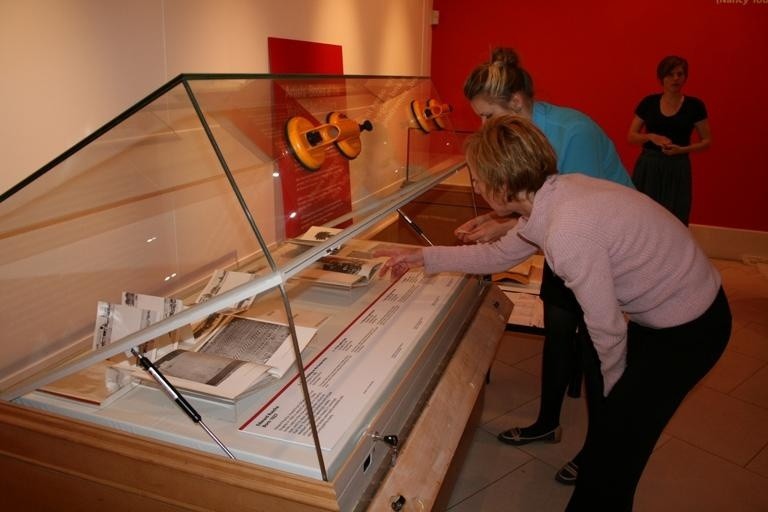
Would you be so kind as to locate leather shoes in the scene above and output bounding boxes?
[554,461,579,486]
[497,422,563,446]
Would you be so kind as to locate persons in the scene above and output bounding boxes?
[384,112,733,511]
[465,45,636,447]
[627,56,716,226]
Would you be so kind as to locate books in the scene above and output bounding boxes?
[288,226,345,252]
[287,255,382,291]
[132,312,317,404]
